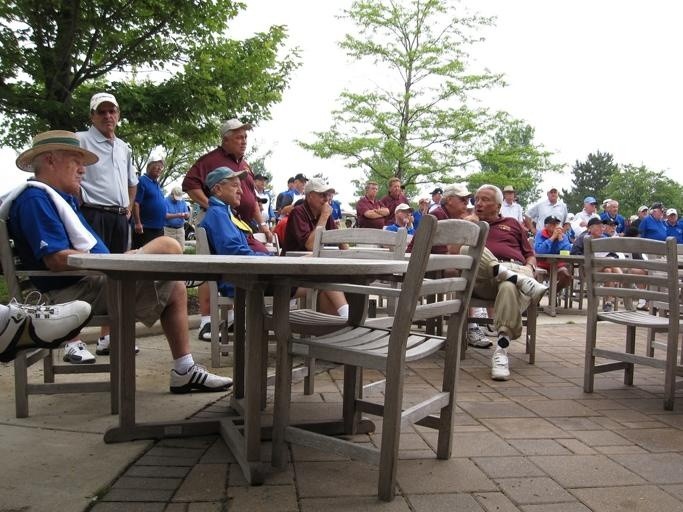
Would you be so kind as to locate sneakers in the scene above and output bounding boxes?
[96,334,140,355]
[63,340,96,363]
[468,312,510,380]
[170,363,233,393]
[603,303,613,312]
[520,276,548,305]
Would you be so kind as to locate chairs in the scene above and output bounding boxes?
[263,225,407,414]
[459,243,536,363]
[194,226,316,370]
[272,213,491,502]
[581,233,682,413]
[646,236,681,358]
[0,217,124,418]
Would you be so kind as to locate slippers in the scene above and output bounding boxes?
[199,323,221,341]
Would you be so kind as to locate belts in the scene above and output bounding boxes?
[85,203,127,215]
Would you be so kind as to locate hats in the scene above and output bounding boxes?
[147,155,163,165]
[16,129,99,171]
[90,93,119,111]
[545,186,678,224]
[395,184,473,211]
[222,119,252,135]
[255,173,335,203]
[503,185,515,192]
[173,186,184,201]
[206,167,248,190]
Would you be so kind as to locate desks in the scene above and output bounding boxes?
[65,252,406,487]
[537,254,586,317]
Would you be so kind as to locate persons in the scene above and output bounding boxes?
[449,184,548,380]
[355,177,683,347]
[165,118,351,342]
[60,92,138,366]
[129,155,164,249]
[2,129,234,395]
